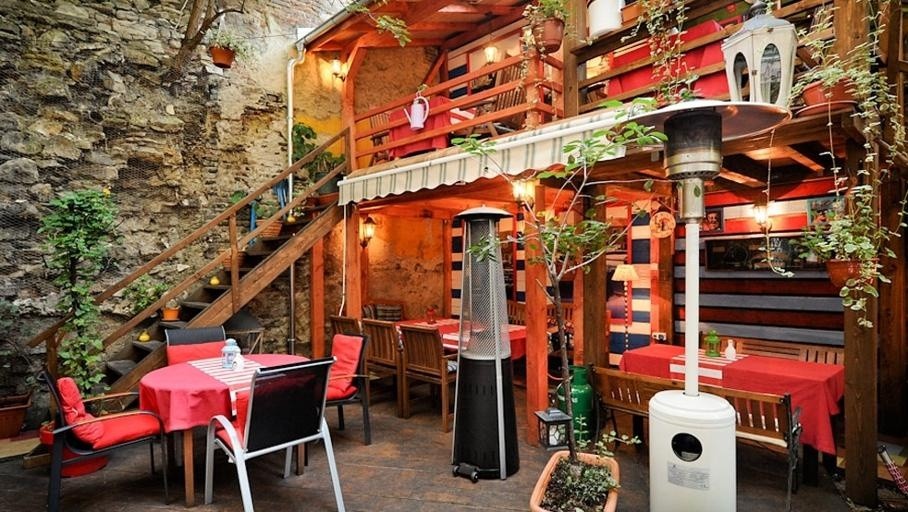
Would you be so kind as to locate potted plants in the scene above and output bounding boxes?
[526,0,586,54]
[620,0,703,106]
[449,97,701,512]
[204,29,254,69]
[157,122,346,322]
[0,188,126,477]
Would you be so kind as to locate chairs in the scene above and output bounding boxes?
[368,50,527,167]
[329,299,575,433]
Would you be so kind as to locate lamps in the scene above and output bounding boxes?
[610,260,639,350]
[329,55,345,81]
[360,217,376,249]
[482,12,498,66]
[533,396,572,451]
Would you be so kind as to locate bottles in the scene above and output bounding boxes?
[427,306,435,325]
[705,331,720,356]
[221,339,241,370]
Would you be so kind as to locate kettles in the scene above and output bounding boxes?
[725,339,736,359]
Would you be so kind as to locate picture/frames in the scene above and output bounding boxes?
[699,208,725,234]
